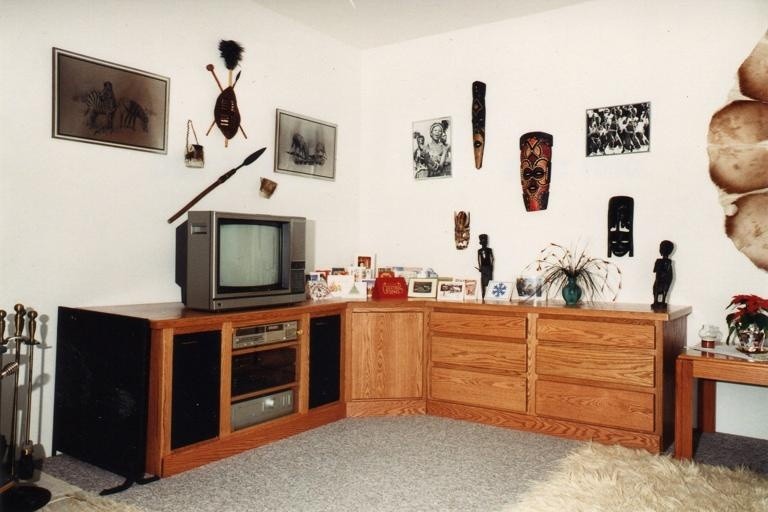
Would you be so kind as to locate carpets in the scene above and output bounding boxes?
[502,438,768,512]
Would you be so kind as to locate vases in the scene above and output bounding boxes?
[736,324,765,353]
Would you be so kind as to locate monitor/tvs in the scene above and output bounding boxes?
[175,210,306,313]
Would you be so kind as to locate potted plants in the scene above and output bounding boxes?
[508,234,623,308]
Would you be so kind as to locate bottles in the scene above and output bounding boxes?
[185,143,206,168]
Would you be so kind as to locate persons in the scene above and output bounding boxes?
[652,239,674,310]
[587,102,650,156]
[417,124,449,177]
[476,234,494,300]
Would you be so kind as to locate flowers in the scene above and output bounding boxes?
[725,294,768,346]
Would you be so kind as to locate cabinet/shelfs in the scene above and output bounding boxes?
[51,298,350,485]
[344,296,431,418]
[425,298,694,456]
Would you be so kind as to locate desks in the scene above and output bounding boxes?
[674,339,768,462]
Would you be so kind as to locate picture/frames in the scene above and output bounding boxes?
[273,107,339,182]
[411,116,453,182]
[51,46,172,155]
[304,253,514,302]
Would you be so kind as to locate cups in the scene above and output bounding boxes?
[702,339,715,348]
[328,274,355,296]
[702,352,714,358]
[259,178,277,199]
